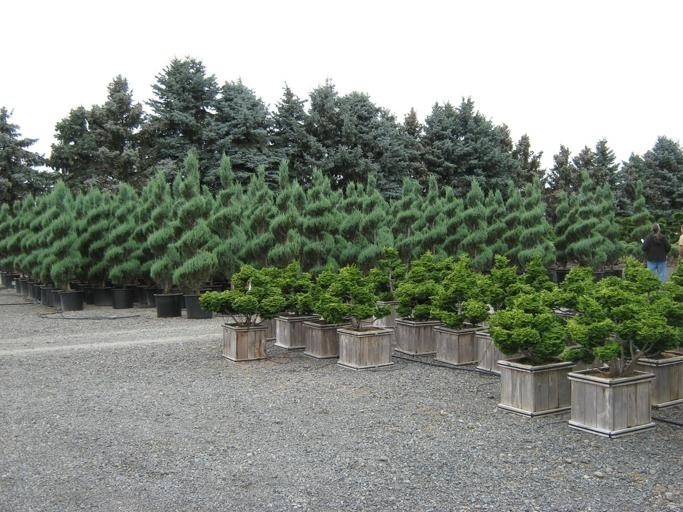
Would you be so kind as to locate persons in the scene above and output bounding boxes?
[679,226,683,257]
[640,223,671,282]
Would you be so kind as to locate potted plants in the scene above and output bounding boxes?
[198,252,682,436]
[1,147,656,320]
